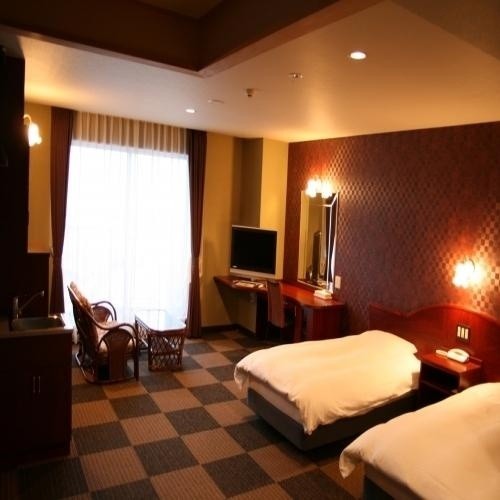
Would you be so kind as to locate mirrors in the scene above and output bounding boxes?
[296,191,339,295]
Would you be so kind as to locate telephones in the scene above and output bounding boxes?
[435,347,471,364]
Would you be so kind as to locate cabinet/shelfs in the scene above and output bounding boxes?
[3,334,73,466]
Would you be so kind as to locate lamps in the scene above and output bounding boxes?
[451,257,478,289]
[302,176,333,200]
[23,114,43,147]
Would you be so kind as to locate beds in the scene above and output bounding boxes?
[234,328,421,452]
[338,382,500,500]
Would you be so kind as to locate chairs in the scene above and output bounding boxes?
[66,280,137,385]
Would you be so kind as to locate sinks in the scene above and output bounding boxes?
[0,317,74,338]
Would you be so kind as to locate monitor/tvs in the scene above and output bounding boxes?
[229,225,278,287]
[306,231,321,283]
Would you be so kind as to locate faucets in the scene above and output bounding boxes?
[11,291,45,320]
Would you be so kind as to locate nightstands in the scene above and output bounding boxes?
[413,344,483,395]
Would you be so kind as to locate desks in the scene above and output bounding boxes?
[214,275,345,343]
[134,311,187,372]
